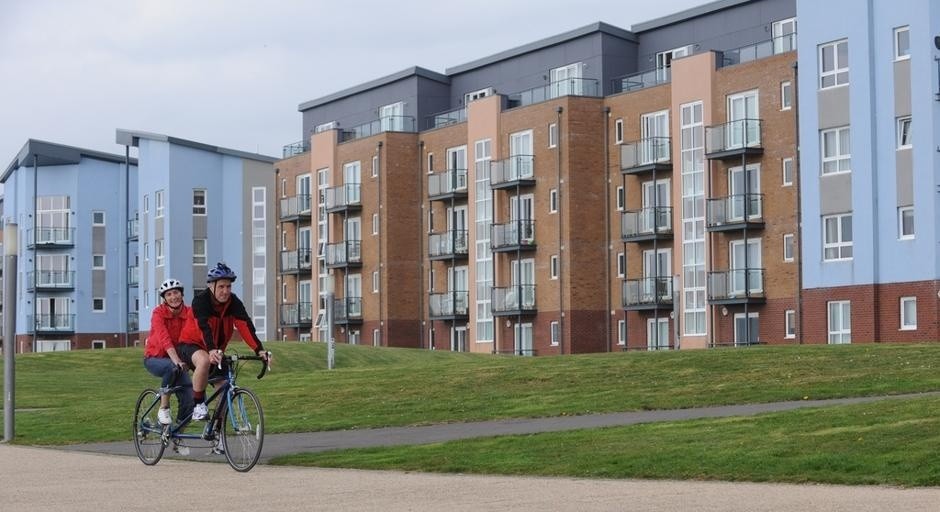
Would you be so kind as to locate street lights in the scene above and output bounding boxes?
[1,222,19,443]
[326,273,337,371]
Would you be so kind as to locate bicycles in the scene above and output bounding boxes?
[130,350,270,474]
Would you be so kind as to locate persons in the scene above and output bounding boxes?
[177,262,273,455]
[141,278,195,457]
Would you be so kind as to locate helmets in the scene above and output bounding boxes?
[157,278,184,296]
[206,262,237,283]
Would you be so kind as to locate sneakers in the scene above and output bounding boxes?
[169,438,191,457]
[157,407,172,425]
[213,430,226,456]
[191,402,210,421]
